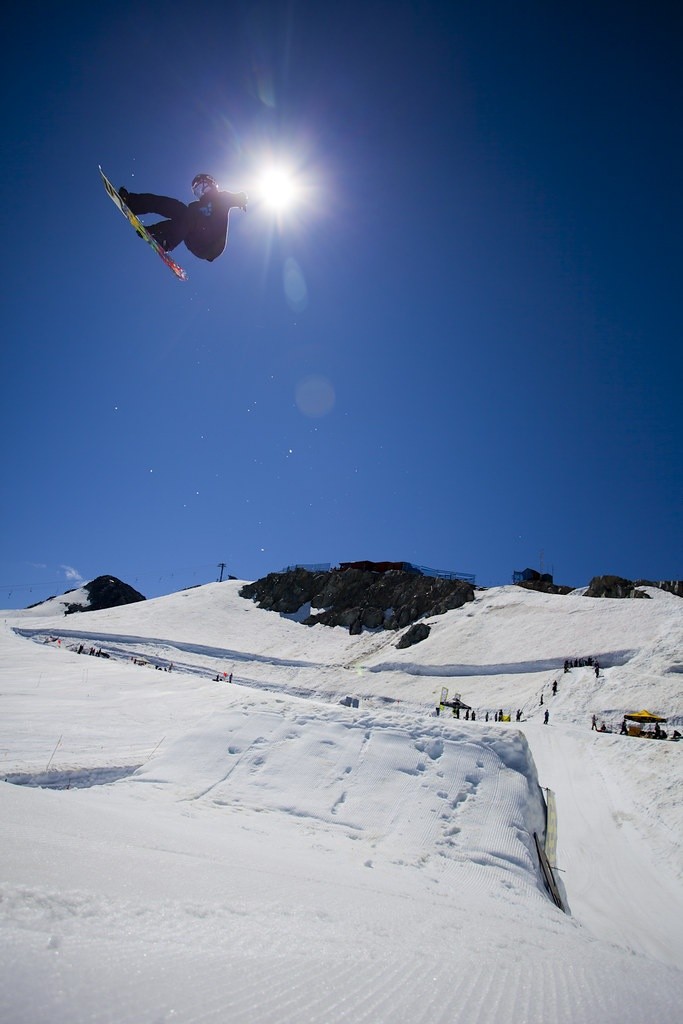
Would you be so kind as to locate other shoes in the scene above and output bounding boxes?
[136,226,166,249]
[119,187,136,217]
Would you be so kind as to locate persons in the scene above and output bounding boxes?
[591,714,599,731]
[77,644,233,683]
[456,707,522,722]
[544,709,549,724]
[594,661,600,678]
[119,174,248,262]
[540,694,544,705]
[564,657,596,673]
[552,680,558,695]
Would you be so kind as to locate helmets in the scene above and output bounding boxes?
[191,174,218,199]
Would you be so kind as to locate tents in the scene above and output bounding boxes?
[624,710,668,733]
[440,698,471,710]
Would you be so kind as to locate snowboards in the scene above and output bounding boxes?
[98,164,190,282]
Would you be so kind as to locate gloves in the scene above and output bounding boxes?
[235,192,248,212]
